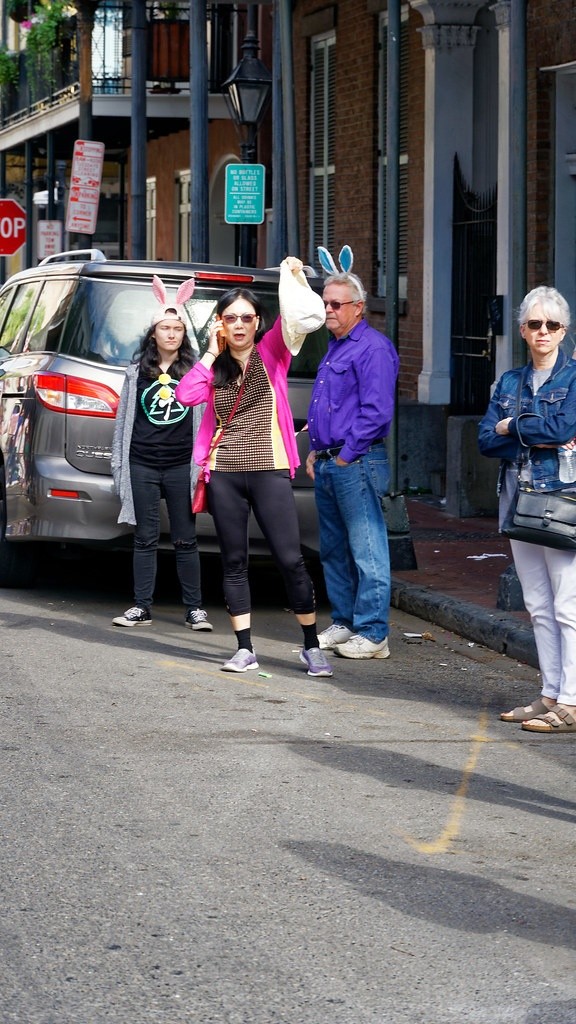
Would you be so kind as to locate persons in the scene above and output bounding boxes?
[112,305,214,631]
[305,272,399,660]
[174,256,334,677]
[477,286,576,733]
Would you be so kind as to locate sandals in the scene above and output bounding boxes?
[521,704,576,733]
[500,698,552,722]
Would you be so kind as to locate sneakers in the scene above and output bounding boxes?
[316,623,354,649]
[221,645,259,673]
[299,645,333,677]
[185,608,213,631]
[112,605,152,626]
[334,634,390,660]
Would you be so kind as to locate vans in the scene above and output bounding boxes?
[0,248,333,588]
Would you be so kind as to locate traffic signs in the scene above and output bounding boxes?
[64,140,107,235]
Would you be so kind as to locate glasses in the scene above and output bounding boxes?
[323,298,363,309]
[221,314,259,324]
[522,319,566,331]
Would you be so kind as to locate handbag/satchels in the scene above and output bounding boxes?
[501,482,576,552]
[192,471,209,514]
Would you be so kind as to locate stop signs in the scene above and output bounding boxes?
[0,199,30,258]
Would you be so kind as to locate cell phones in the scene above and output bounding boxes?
[216,314,226,352]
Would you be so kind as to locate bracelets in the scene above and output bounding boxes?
[207,350,217,358]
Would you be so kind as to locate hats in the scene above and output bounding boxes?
[149,273,195,325]
[279,258,326,356]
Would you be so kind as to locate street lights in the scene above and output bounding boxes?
[219,31,273,266]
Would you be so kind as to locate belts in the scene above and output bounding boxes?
[317,436,386,461]
